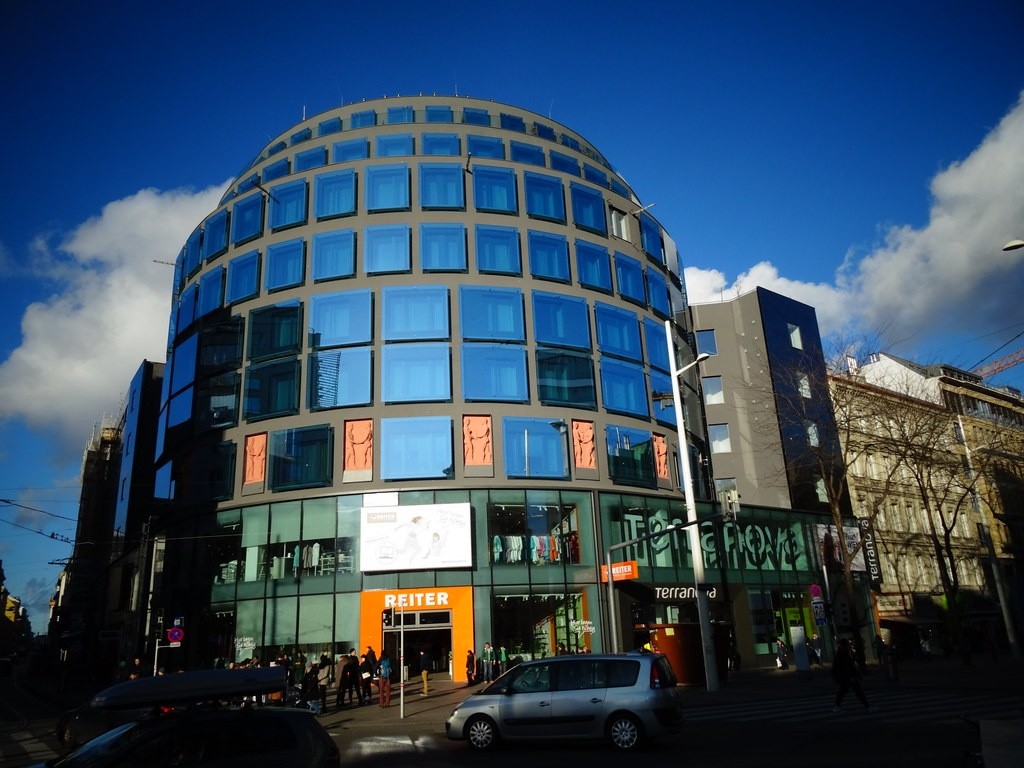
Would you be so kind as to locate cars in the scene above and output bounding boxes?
[445,652,685,752]
[55,682,231,748]
[24,704,341,768]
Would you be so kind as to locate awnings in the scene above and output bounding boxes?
[881,616,944,624]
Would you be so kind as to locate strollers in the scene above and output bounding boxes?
[293,674,322,715]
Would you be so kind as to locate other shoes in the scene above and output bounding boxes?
[368,699,371,703]
[317,708,321,716]
[384,704,389,708]
[342,703,346,706]
[490,680,492,682]
[336,703,340,706]
[420,693,427,696]
[484,680,487,684]
[349,700,352,703]
[376,704,382,707]
[320,707,327,713]
[357,698,363,705]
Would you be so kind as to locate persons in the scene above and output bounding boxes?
[833,639,880,714]
[481,642,496,683]
[419,648,429,696]
[498,644,509,675]
[805,633,822,665]
[466,650,474,686]
[558,643,591,655]
[777,640,789,670]
[875,635,884,669]
[115,647,393,713]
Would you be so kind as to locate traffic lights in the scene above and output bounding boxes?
[383,609,391,625]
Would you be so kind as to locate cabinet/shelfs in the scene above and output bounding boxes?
[318,556,354,576]
